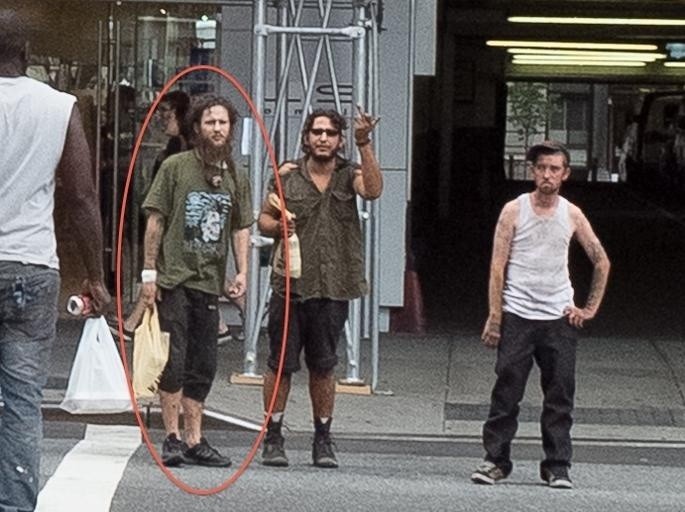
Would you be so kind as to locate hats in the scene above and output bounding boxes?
[526,138,571,160]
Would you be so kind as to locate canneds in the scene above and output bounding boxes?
[67,294,94,317]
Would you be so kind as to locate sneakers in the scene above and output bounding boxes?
[470,457,509,485]
[538,460,574,489]
[159,435,183,466]
[182,438,232,468]
[310,416,339,468]
[262,413,289,467]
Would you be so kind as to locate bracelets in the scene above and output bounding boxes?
[355,138,370,147]
[139,267,157,284]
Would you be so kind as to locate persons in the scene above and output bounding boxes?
[468,137,615,489]
[1,8,113,511]
[255,101,386,472]
[139,89,256,470]
[151,90,191,180]
